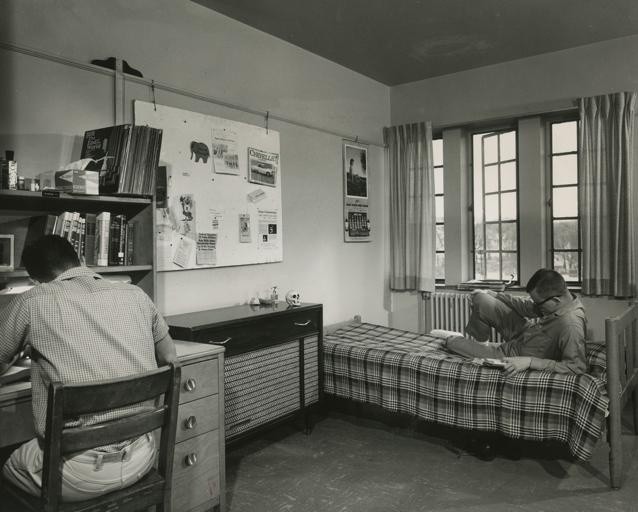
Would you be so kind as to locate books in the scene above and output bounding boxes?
[79,124,165,198]
[19,212,136,268]
[0,358,31,386]
[472,357,506,369]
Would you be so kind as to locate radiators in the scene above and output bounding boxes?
[418,290,512,349]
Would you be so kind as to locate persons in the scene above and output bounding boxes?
[2,233,178,507]
[348,158,356,183]
[429,268,588,381]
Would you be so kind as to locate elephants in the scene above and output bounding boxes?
[189,140,210,164]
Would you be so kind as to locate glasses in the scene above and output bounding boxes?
[533,293,562,310]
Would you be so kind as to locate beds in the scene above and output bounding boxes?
[324,297,638,492]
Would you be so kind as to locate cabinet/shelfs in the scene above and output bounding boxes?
[166,300,326,466]
[0,340,226,512]
[0,186,160,311]
